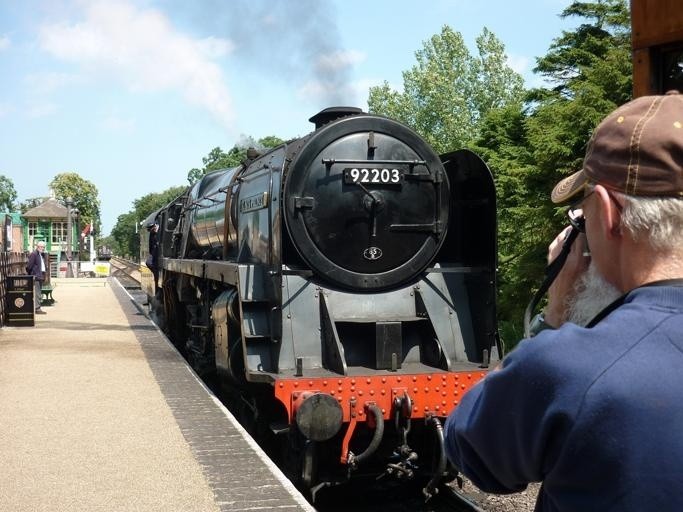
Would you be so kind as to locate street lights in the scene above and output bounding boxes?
[65,195,74,277]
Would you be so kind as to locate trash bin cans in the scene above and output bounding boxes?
[6,276,34,326]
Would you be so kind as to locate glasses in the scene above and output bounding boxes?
[566,189,621,231]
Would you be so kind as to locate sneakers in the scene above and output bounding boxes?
[35,310,45,315]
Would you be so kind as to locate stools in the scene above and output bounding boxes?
[40,289,55,306]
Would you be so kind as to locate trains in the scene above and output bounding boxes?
[97,244,111,261]
[139,107,505,512]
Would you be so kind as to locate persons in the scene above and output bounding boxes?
[145,223,159,296]
[26,241,47,314]
[443,91,683,512]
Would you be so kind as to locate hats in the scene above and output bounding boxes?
[551,91,682,203]
